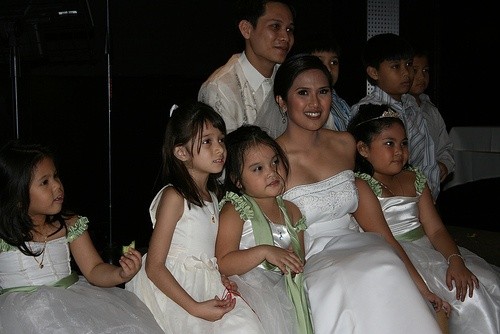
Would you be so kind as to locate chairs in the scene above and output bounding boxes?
[445,127,500,189]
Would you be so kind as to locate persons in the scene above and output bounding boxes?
[125,102,265,334]
[408,54,456,181]
[351,33,440,204]
[0,142,164,334]
[305,40,351,131]
[346,102,500,334]
[198,0,336,140]
[273,54,451,334]
[214,124,315,334]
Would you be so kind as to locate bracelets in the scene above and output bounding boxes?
[447,253,464,265]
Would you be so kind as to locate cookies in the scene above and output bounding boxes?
[122,240,135,255]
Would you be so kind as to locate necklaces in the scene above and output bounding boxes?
[378,178,399,198]
[199,190,216,224]
[28,223,47,269]
[255,198,283,240]
